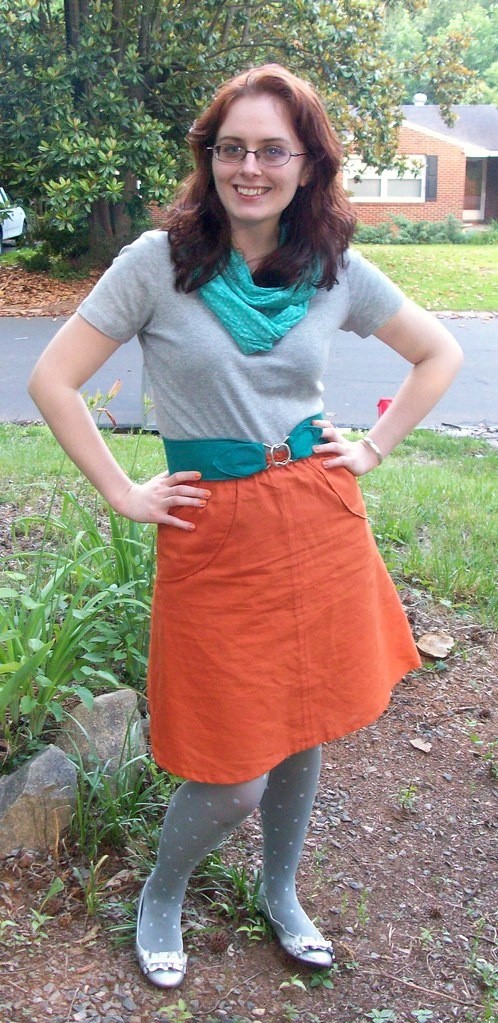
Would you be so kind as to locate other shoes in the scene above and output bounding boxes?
[257,883,335,968]
[134,872,189,988]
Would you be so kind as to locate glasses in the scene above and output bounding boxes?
[206,145,310,167]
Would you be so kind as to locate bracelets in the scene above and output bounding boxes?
[362,433,385,466]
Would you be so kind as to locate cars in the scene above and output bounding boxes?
[0,187,29,255]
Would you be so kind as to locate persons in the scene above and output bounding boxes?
[26,63,466,987]
